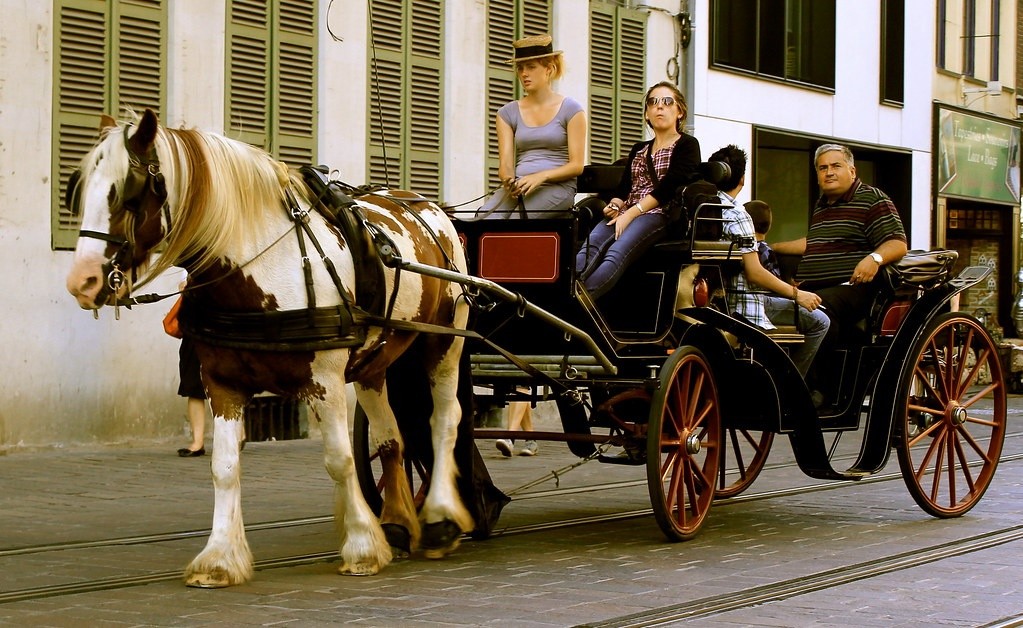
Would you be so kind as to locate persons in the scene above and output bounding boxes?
[495,385,539,458]
[576,81,702,301]
[770,144,907,382]
[479,34,587,219]
[709,145,831,408]
[177,280,245,457]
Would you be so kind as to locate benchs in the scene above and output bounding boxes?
[577,164,743,261]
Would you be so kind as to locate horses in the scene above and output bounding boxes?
[65,108,471,588]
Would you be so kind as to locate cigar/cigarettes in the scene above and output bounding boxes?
[819,304,826,309]
[856,275,860,278]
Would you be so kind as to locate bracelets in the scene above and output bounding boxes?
[791,278,805,300]
[611,201,620,208]
[636,202,644,214]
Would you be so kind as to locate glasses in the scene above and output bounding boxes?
[645,96,677,107]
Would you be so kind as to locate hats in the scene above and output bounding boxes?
[504,35,562,63]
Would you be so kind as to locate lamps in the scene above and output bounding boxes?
[959,74,1002,99]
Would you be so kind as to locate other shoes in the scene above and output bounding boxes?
[812,383,837,407]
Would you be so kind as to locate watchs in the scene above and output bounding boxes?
[869,252,883,266]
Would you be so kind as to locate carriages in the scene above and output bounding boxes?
[65,109,1007,589]
